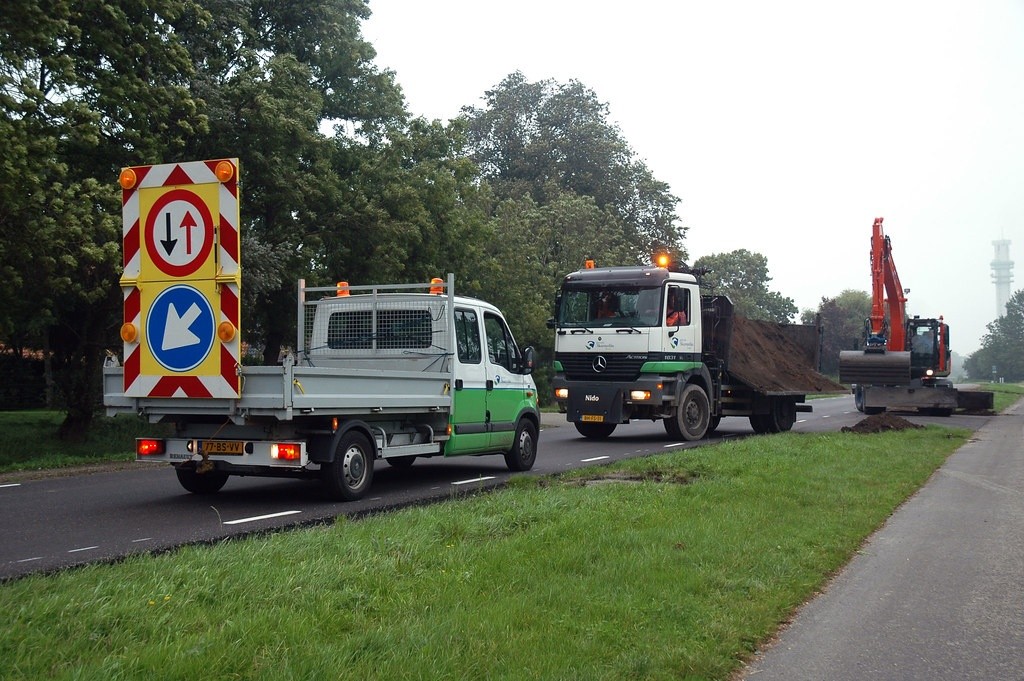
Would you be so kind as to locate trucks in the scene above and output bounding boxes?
[544,256,825,442]
[102,155,542,503]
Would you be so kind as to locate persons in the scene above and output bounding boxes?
[598,293,618,318]
[340,316,366,337]
[922,327,939,346]
[645,293,686,326]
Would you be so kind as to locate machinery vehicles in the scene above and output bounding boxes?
[837,217,995,418]
